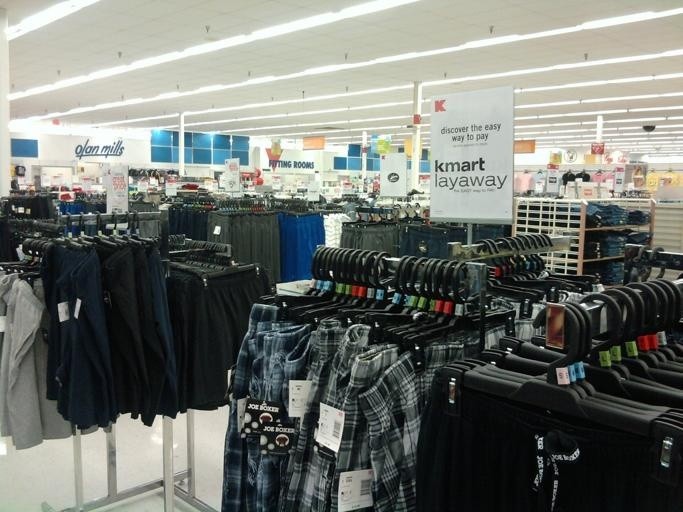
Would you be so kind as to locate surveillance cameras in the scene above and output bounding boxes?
[644,126,655,132]
